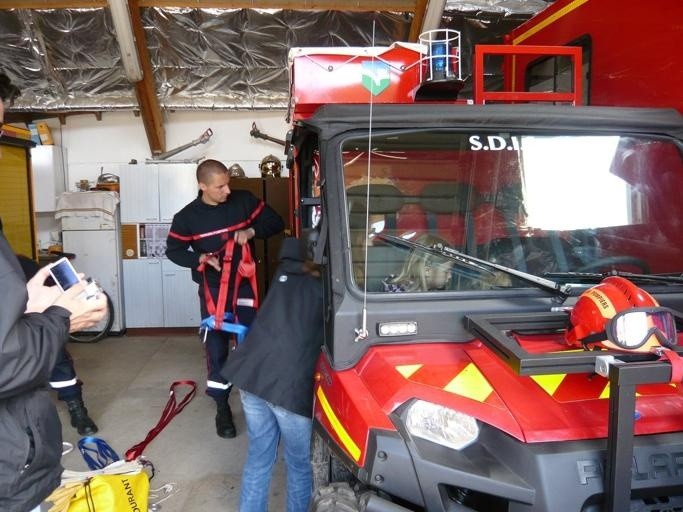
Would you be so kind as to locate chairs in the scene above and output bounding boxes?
[347,182,571,292]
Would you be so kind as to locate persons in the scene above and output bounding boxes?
[15,255,98,437]
[0,209,108,512]
[220,199,365,512]
[164,158,286,437]
[378,233,456,291]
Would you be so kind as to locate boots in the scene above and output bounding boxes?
[67,397,99,436]
[215,403,236,438]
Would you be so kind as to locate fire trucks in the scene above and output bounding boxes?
[280,28,683,510]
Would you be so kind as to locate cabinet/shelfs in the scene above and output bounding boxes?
[122,259,202,336]
[30,144,68,213]
[228,178,289,308]
[119,163,201,224]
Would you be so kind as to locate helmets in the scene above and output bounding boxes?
[567,276,678,354]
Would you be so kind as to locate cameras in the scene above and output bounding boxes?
[50,258,101,305]
